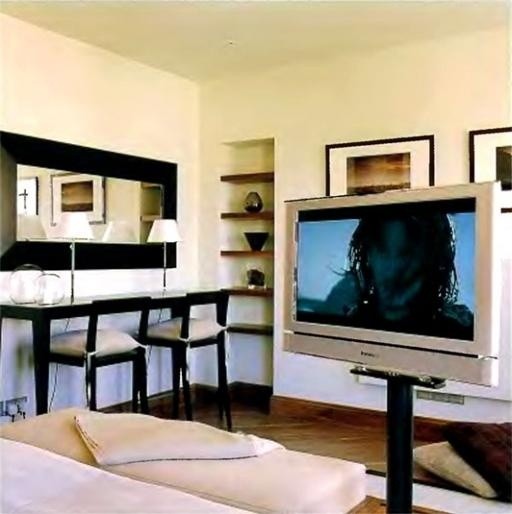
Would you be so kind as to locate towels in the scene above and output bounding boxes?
[73,413,288,466]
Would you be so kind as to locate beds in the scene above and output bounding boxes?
[0,409,367,514]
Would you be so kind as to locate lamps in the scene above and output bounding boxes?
[145,217,186,296]
[49,209,96,301]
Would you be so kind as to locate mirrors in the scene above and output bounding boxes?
[0,131,176,268]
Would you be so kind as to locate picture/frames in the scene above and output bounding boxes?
[14,169,108,222]
[324,128,512,211]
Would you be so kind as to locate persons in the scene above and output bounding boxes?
[336,214,472,339]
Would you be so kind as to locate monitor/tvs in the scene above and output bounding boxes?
[282,180,502,390]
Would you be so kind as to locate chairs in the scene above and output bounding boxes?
[47,288,235,429]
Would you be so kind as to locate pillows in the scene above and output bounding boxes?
[413,420,511,502]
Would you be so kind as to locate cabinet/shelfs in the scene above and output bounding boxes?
[218,173,273,334]
[140,181,163,221]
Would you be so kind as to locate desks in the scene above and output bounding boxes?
[0,290,197,415]
